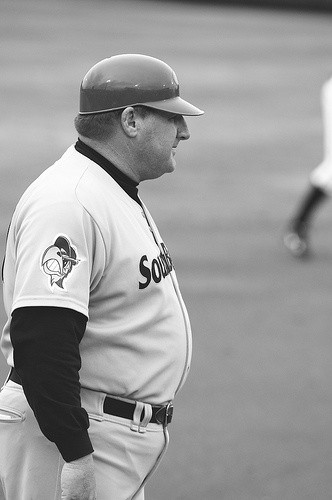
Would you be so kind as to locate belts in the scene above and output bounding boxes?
[8,366,174,426]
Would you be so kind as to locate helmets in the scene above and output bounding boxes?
[80,53,204,116]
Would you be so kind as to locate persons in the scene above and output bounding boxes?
[0,54,204,500]
[284,78,332,257]
